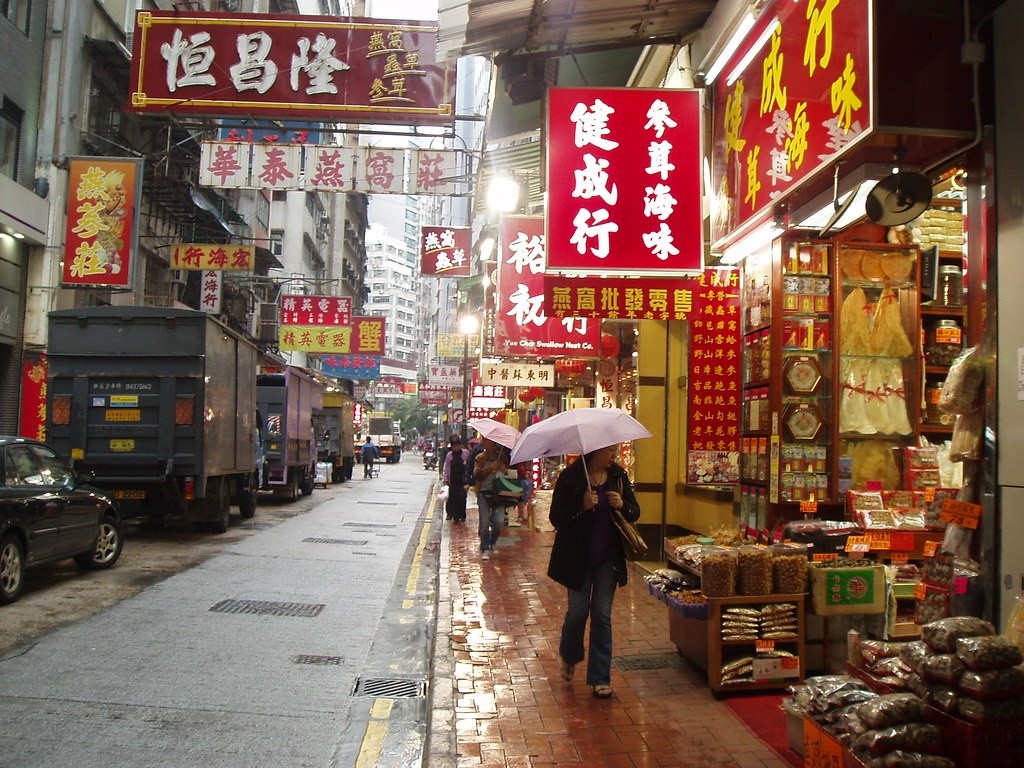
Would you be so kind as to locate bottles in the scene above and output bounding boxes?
[925,381,956,426]
[926,320,962,365]
[933,265,964,309]
[700,542,809,599]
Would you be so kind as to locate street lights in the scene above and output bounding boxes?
[458,314,478,445]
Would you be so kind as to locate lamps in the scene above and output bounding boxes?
[691,0,777,85]
[499,56,560,105]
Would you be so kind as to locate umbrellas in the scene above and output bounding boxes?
[470,418,522,474]
[510,408,655,492]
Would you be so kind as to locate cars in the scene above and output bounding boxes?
[0,434,124,605]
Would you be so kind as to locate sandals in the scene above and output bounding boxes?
[593,684,611,697]
[561,659,574,681]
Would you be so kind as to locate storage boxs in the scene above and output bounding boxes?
[805,558,886,616]
[644,575,709,619]
[782,664,1024,768]
[784,355,825,441]
[856,530,958,639]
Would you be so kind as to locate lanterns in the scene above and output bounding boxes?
[601,332,620,360]
[519,387,545,404]
[555,359,585,408]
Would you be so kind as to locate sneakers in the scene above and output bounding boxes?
[482,549,489,560]
[491,545,498,554]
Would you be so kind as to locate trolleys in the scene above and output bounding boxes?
[367,458,380,478]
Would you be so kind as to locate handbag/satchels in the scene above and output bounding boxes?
[492,468,523,505]
[610,475,649,562]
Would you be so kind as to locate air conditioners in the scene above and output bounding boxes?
[246,303,281,341]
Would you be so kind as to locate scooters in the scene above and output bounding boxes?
[425,449,438,471]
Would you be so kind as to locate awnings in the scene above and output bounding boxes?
[438,0,720,142]
[85,36,247,234]
[474,141,545,214]
[257,246,284,269]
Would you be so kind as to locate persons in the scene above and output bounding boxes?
[439,434,532,560]
[548,444,640,697]
[361,436,379,478]
[418,440,438,466]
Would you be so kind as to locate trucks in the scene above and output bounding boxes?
[354,411,402,464]
[45,305,268,533]
[256,367,361,503]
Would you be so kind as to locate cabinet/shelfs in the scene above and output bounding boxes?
[665,556,811,701]
[861,549,939,641]
[737,195,966,540]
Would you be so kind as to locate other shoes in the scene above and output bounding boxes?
[488,527,491,531]
[508,521,521,526]
[446,513,465,524]
